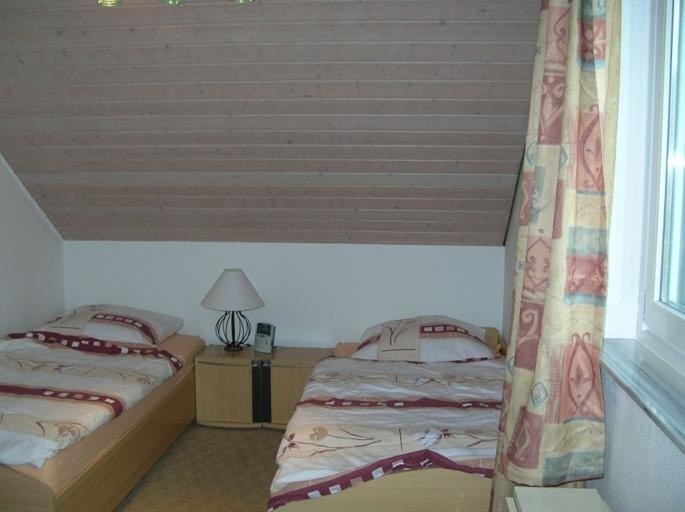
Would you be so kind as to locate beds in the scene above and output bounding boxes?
[1,296,207,512]
[270,318,594,512]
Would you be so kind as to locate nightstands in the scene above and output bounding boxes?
[263,347,338,432]
[191,342,265,433]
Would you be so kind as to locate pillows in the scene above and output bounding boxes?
[34,300,186,348]
[351,314,504,363]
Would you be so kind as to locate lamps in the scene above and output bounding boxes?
[198,265,264,354]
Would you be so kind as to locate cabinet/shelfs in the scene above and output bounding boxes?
[499,482,613,512]
